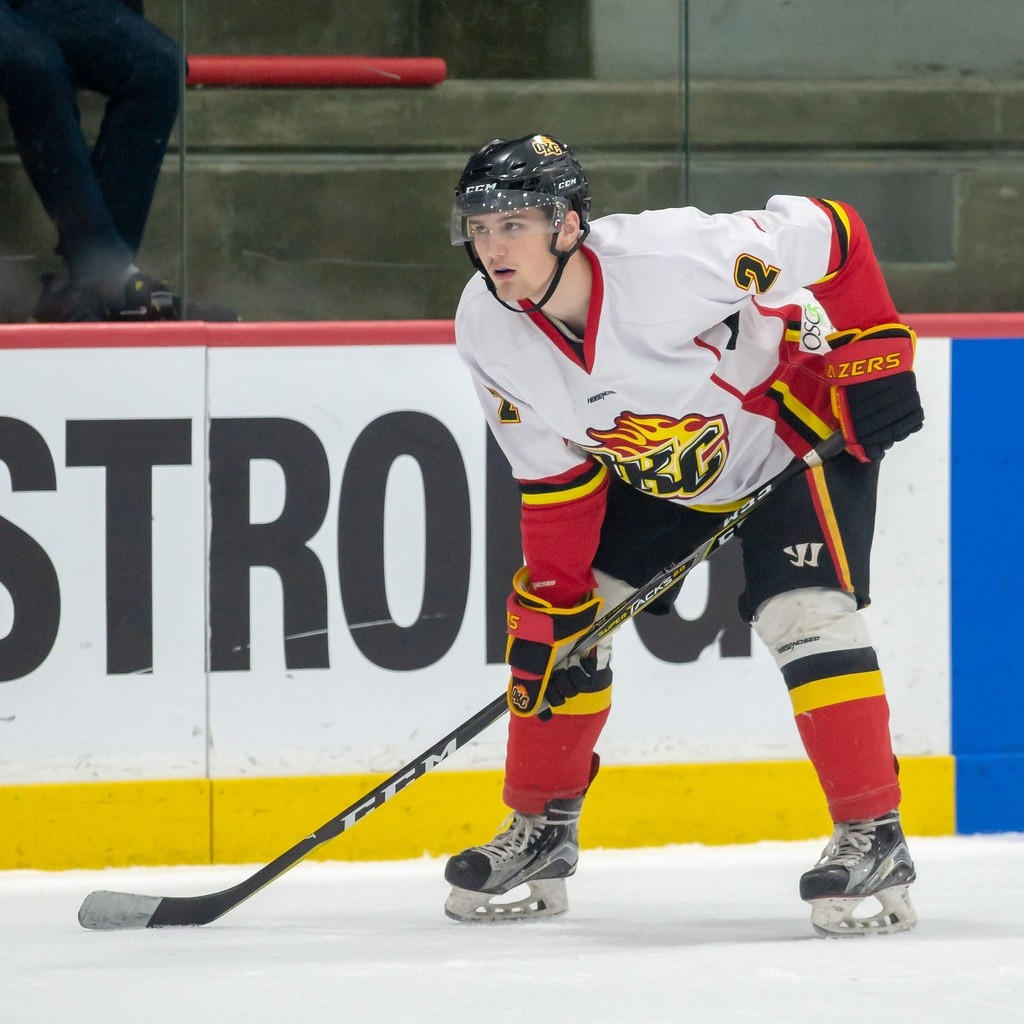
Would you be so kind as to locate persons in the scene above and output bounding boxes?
[447,132,921,937]
[0,0,206,322]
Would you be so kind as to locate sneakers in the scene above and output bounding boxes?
[799,753,914,935]
[33,272,106,321]
[104,271,240,322]
[443,751,601,922]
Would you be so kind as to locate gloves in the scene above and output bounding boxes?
[505,564,612,722]
[822,323,925,463]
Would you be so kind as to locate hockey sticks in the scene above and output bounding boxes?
[74,428,849,934]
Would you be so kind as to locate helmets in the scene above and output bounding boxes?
[453,133,590,229]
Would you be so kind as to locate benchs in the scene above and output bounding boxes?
[180,55,449,89]
